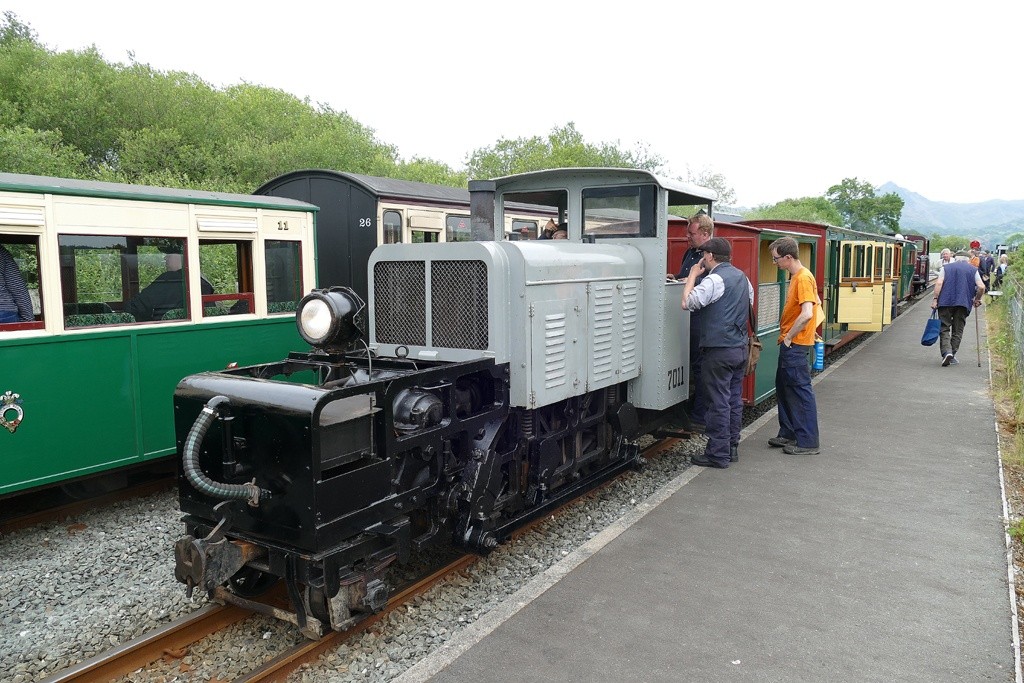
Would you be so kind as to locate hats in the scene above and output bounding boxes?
[698,238,730,257]
[157,239,184,254]
[551,223,567,235]
[953,252,971,258]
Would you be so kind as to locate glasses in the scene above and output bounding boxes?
[772,256,783,263]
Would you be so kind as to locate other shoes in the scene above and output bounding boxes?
[941,352,959,367]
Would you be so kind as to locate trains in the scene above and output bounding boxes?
[173,169,930,643]
[0,167,689,501]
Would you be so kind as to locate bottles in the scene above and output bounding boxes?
[813,338,824,369]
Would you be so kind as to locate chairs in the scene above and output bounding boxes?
[65,300,299,328]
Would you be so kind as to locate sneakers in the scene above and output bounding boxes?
[768,434,797,447]
[783,442,821,454]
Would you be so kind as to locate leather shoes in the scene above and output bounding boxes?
[691,454,729,468]
[730,447,738,462]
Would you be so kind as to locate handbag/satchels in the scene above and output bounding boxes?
[921,307,941,346]
[815,301,826,328]
[744,331,763,376]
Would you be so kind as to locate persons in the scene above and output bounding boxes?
[666,214,714,434]
[968,249,995,293]
[768,236,821,455]
[115,240,216,321]
[987,254,1009,305]
[537,218,568,240]
[682,237,754,469]
[931,250,986,367]
[933,248,955,276]
[0,244,36,323]
